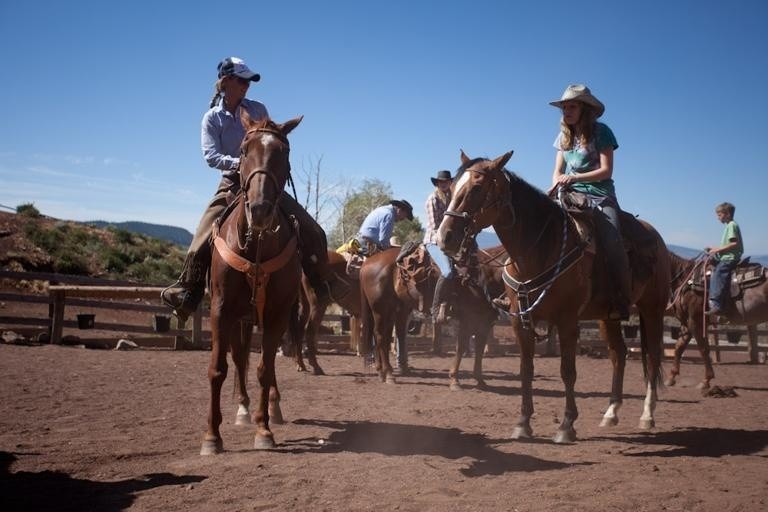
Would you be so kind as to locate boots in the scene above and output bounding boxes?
[602,268,634,321]
[493,295,511,310]
[300,258,351,308]
[162,251,207,313]
[432,274,454,323]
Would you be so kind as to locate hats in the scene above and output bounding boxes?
[548,85,605,118]
[217,57,260,82]
[432,172,452,187]
[389,198,412,221]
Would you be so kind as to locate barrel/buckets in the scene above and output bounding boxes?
[407,316,423,335]
[623,324,638,338]
[152,312,173,332]
[76,313,97,330]
[727,328,742,344]
[339,315,350,332]
[671,326,681,340]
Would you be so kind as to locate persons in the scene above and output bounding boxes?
[355,200,413,257]
[161,55,329,321]
[549,85,629,320]
[704,202,744,315]
[423,171,456,324]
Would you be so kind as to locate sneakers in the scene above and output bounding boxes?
[704,306,727,315]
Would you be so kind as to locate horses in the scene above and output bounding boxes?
[358,244,510,393]
[667,249,768,391]
[434,147,671,446]
[198,106,305,459]
[295,246,413,377]
